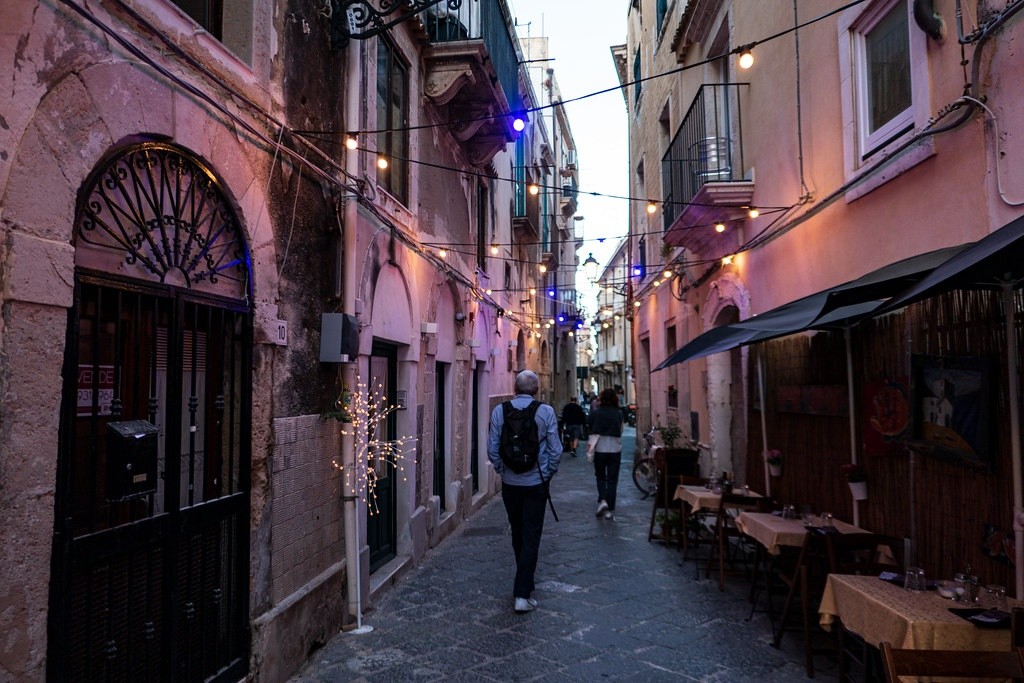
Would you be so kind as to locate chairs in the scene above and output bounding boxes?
[687,136,732,190]
[678,477,1024,683]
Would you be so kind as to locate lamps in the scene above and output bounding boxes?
[582,253,628,298]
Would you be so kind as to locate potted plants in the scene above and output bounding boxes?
[657,423,682,450]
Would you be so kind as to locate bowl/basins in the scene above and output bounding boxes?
[934,580,955,598]
[800,513,816,524]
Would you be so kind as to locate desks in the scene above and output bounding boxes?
[818,573,1024,683]
[734,510,873,647]
[673,485,771,583]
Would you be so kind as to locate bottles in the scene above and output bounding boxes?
[719,471,728,495]
[791,505,796,519]
[820,512,832,527]
[782,504,790,520]
[954,567,981,603]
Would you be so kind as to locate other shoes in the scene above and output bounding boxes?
[514,597,537,614]
[570,448,577,457]
[605,512,612,519]
[596,502,608,516]
[498,399,553,474]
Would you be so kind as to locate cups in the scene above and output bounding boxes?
[903,567,926,598]
[982,584,1009,612]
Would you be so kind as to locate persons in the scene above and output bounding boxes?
[582,389,627,424]
[562,396,585,457]
[588,389,624,519]
[487,369,564,612]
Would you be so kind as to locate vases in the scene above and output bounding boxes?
[768,464,782,476]
[848,481,870,501]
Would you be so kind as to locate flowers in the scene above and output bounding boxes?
[841,462,868,481]
[761,448,783,466]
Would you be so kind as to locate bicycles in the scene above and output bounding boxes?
[631,425,680,495]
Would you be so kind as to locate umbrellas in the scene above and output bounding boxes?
[727,243,975,529]
[850,214,1024,601]
[650,296,896,499]
